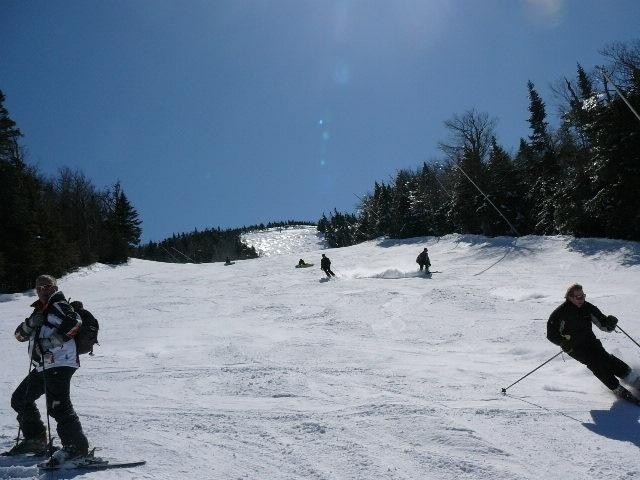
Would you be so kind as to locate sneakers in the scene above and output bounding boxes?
[616,376,640,402]
[10,430,48,454]
[49,438,89,464]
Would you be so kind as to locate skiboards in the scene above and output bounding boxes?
[418,272,442,274]
[620,378,640,406]
[0,451,146,471]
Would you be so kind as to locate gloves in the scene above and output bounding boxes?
[605,314,619,327]
[22,312,43,336]
[561,340,574,352]
[36,333,63,354]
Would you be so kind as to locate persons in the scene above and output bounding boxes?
[321,253,336,278]
[415,247,432,275]
[223,255,231,264]
[546,283,639,406]
[10,273,90,468]
[298,258,306,265]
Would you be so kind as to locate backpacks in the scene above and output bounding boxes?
[45,294,99,354]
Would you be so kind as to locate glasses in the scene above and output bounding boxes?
[36,284,55,292]
[570,294,587,300]
